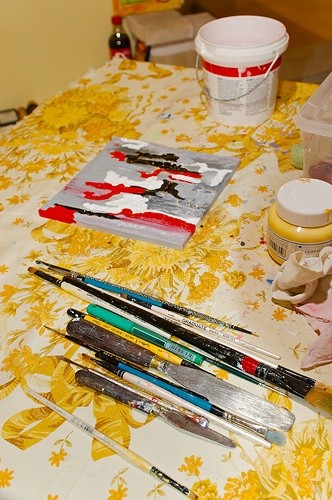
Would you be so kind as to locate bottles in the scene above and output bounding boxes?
[110,15,132,60]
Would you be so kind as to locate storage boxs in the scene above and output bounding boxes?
[296,70,332,185]
[123,9,195,58]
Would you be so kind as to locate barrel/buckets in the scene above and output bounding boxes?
[195,15,289,127]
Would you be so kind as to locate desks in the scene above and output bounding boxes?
[0,58,332,500]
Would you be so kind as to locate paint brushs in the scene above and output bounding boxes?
[27,388,196,500]
[29,260,332,450]
